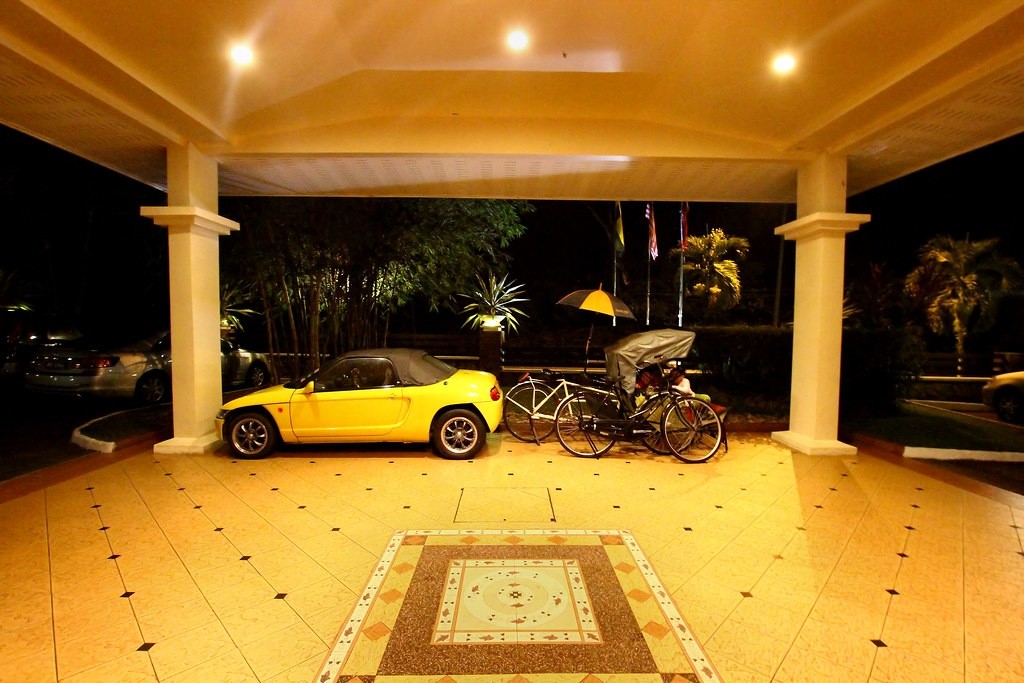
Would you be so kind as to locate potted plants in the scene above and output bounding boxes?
[456,273,532,337]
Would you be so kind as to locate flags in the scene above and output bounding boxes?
[682,202,689,247]
[616,202,624,252]
[645,201,659,260]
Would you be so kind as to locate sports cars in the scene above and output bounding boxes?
[214,348,504,460]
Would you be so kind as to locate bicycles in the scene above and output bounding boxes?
[503,367,637,456]
[552,353,723,464]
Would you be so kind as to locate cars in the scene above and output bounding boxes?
[982,369,1024,425]
[0,303,87,390]
[16,322,273,409]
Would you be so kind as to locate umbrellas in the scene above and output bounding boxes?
[555,283,637,373]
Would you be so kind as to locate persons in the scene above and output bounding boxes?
[635,364,696,404]
